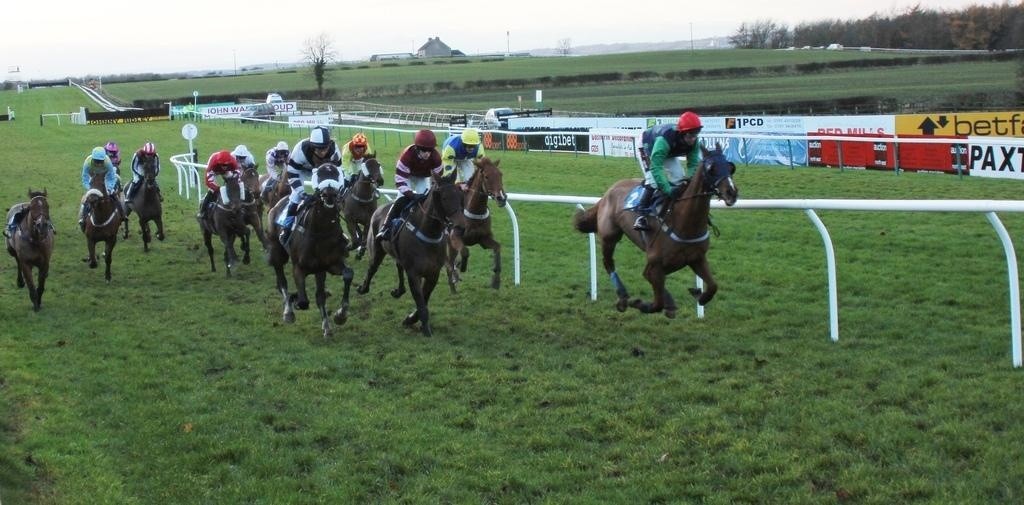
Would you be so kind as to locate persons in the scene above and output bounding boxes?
[180,101,195,120]
[341,133,372,190]
[375,129,443,241]
[633,112,703,231]
[229,144,256,178]
[279,125,342,245]
[260,141,291,200]
[441,129,485,185]
[197,150,242,218]
[78,147,129,225]
[124,142,163,202]
[103,142,121,176]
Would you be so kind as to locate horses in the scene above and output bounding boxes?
[356,164,470,339]
[4,187,54,311]
[336,150,384,261]
[445,157,507,295]
[82,168,122,285]
[573,142,737,319]
[197,162,292,280]
[122,164,163,253]
[267,157,353,338]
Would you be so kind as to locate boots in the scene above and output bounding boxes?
[633,187,654,224]
[125,182,135,201]
[199,190,212,217]
[376,204,401,238]
[279,202,298,238]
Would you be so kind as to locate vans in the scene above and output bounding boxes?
[267,93,282,103]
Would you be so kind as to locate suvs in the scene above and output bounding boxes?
[238,103,276,123]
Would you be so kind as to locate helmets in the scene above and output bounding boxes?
[217,144,250,166]
[308,126,332,148]
[415,129,436,148]
[352,133,367,147]
[462,128,481,145]
[107,142,117,151]
[92,147,106,161]
[276,141,289,152]
[679,112,702,131]
[144,142,154,153]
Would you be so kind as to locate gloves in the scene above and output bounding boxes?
[248,163,254,168]
[667,186,680,199]
[412,194,426,202]
[301,193,316,205]
[351,174,359,183]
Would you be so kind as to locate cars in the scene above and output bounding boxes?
[800,45,812,50]
[483,106,519,128]
[826,43,845,51]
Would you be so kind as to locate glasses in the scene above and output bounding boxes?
[421,147,433,152]
